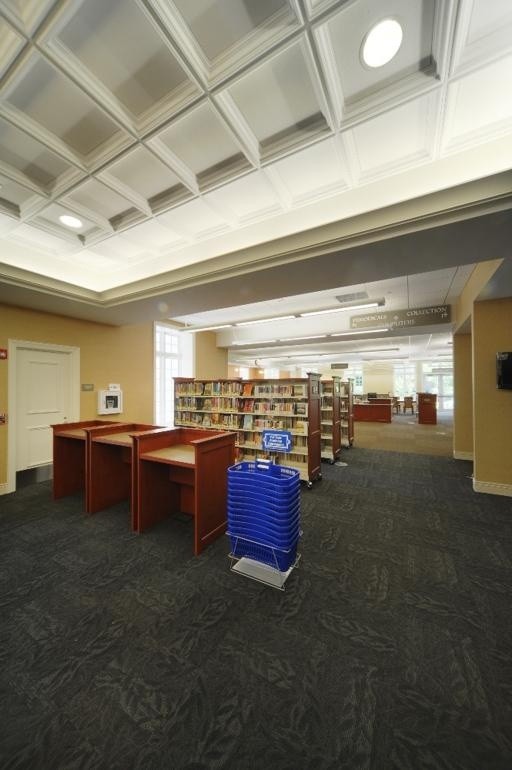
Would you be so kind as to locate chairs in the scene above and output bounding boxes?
[354,392,414,423]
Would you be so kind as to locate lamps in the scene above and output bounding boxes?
[175,293,401,360]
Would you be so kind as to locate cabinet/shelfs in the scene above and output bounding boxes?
[172,373,358,487]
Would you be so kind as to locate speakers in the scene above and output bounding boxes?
[496,352,512,390]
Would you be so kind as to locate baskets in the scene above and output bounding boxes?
[227,462,301,572]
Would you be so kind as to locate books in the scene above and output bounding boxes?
[173,383,348,465]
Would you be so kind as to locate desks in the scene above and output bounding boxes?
[47,417,239,557]
